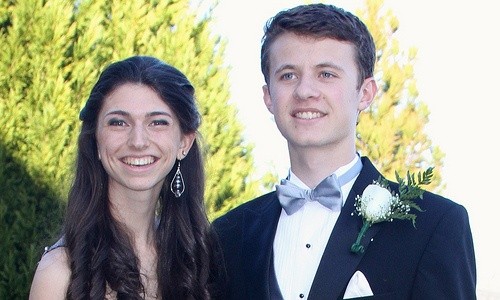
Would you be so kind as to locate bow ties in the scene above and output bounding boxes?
[274,158,364,216]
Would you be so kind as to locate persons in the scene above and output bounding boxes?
[28,57,219,300]
[208,4,477,300]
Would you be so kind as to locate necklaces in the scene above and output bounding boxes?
[138,258,158,298]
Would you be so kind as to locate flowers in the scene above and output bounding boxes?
[346,165,435,252]
